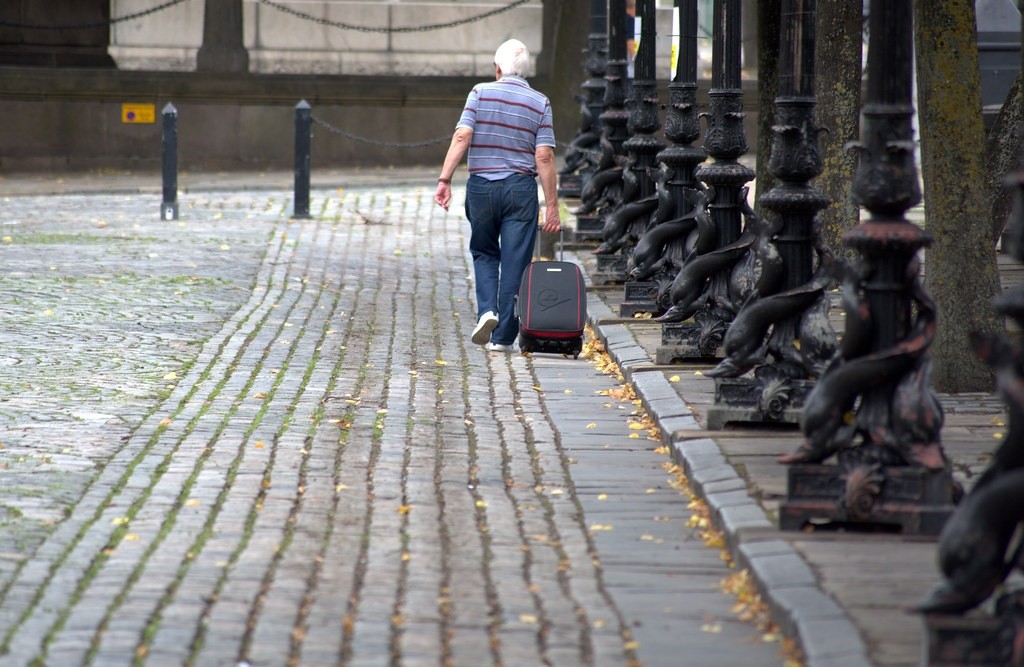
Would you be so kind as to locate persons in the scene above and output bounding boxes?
[435,37,562,352]
[625,1,636,58]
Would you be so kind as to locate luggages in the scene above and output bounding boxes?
[517,225,587,358]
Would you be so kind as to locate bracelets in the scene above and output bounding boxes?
[437,178,452,184]
